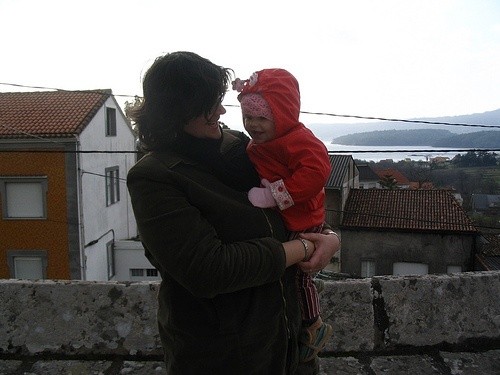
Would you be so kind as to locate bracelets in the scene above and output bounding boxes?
[298,237,308,263]
[325,231,339,251]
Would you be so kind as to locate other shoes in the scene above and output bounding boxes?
[301,315,333,362]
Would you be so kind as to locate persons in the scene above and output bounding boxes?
[127,52,340,375]
[232,69,332,363]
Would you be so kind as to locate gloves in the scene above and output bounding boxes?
[248,178,276,208]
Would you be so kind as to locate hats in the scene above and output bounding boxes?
[241,93,273,122]
